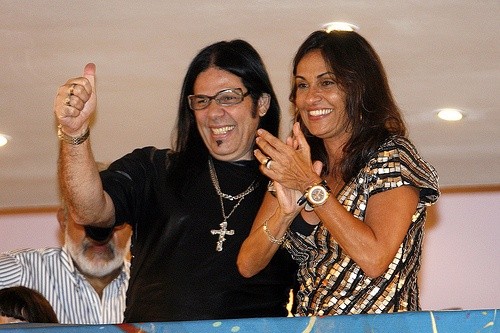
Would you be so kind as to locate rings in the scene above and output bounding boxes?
[262,157,273,169]
[66,94,71,106]
[70,84,76,95]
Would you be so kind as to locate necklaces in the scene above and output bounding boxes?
[206,156,259,251]
[305,176,343,212]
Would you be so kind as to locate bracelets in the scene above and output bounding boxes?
[263,213,287,244]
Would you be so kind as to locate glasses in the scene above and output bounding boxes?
[188,87,251,110]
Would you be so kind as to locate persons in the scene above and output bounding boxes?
[236,30,442,318]
[0,162,133,325]
[54,39,299,324]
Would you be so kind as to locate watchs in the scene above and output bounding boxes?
[58,124,91,145]
[298,180,331,207]
